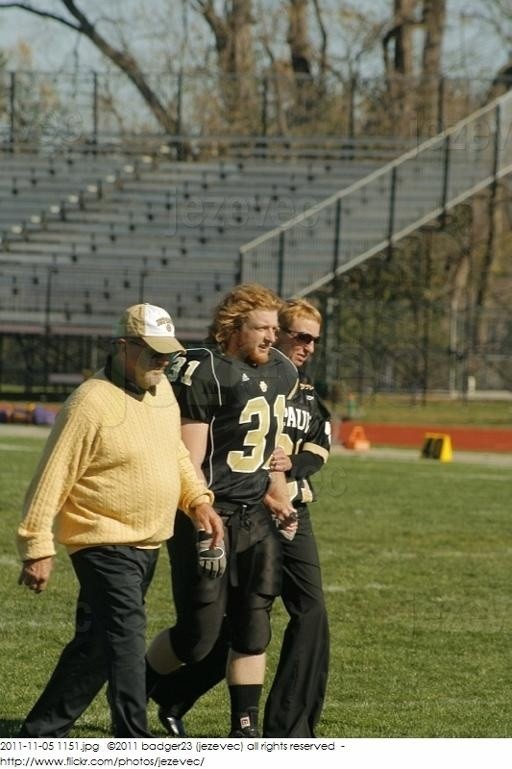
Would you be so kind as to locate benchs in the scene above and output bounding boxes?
[1,138,512,341]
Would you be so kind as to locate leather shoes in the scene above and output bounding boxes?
[139,656,188,737]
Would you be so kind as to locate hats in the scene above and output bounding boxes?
[108,301,186,358]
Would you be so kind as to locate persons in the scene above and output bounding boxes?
[104,279,299,737]
[14,301,227,737]
[152,296,334,737]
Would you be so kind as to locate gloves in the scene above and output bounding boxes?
[193,527,228,580]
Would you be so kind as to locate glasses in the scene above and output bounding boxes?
[280,326,323,347]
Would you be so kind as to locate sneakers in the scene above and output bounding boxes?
[223,713,261,737]
